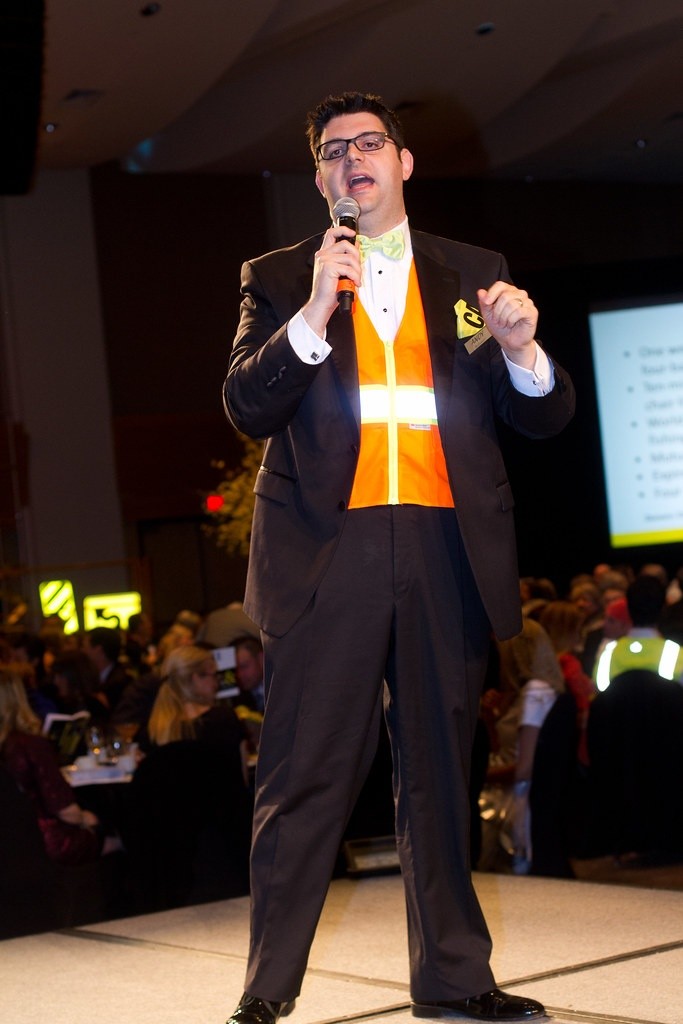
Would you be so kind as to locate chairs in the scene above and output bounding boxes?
[0,756,102,938]
[528,689,584,880]
[123,735,252,913]
[587,669,683,868]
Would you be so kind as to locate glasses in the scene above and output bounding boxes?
[316,132,400,165]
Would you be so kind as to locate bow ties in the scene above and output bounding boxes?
[354,228,406,263]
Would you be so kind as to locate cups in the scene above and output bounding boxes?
[85,727,104,755]
[111,736,127,756]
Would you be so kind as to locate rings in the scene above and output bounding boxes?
[515,298,523,306]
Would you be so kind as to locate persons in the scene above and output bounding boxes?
[0,563,683,915]
[220,89,575,1024]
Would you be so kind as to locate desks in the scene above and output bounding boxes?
[62,751,147,881]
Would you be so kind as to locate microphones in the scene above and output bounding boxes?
[333,196,361,314]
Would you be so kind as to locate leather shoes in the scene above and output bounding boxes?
[412,988,545,1023]
[226,991,295,1024]
